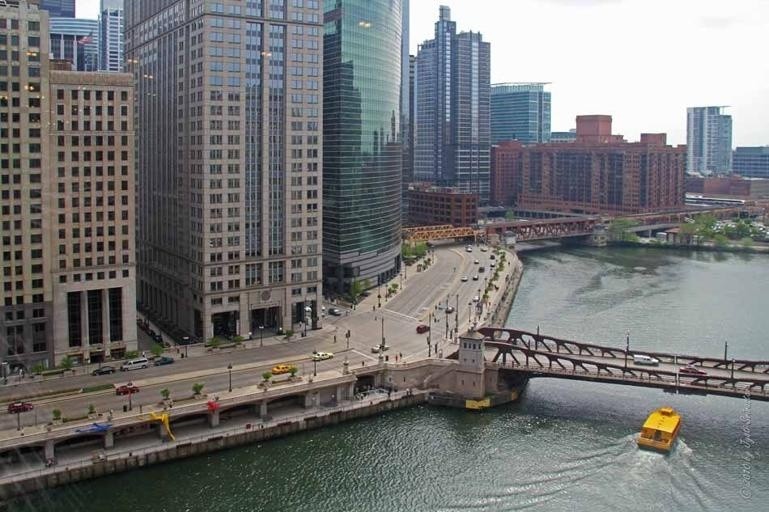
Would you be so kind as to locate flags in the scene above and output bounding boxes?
[78,32,93,46]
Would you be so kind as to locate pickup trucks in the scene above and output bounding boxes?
[633,353,659,365]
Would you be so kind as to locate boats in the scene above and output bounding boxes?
[637,405,682,453]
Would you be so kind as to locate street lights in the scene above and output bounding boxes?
[184,336,189,358]
[13,402,22,431]
[725,339,728,360]
[227,363,234,391]
[731,357,735,377]
[126,383,134,410]
[2,362,9,383]
[625,329,630,351]
[96,347,102,369]
[260,326,264,347]
[313,351,317,376]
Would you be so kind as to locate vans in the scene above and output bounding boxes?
[120,358,149,371]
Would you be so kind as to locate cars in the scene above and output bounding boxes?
[136,319,170,349]
[328,307,342,316]
[322,305,326,317]
[8,402,34,414]
[116,383,140,395]
[445,306,455,314]
[461,244,495,282]
[679,365,707,376]
[272,364,296,374]
[372,344,391,353]
[312,352,334,362]
[93,365,116,375]
[416,324,430,334]
[473,295,480,302]
[154,356,175,366]
[305,304,312,312]
